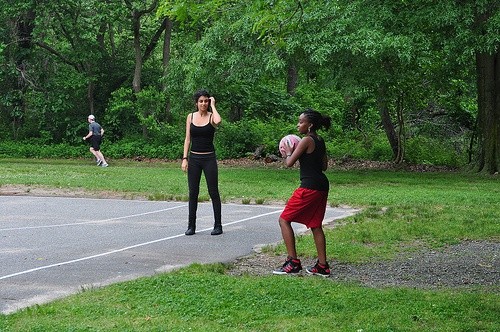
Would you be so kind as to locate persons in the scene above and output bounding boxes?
[83,115,108,167]
[182,92,221,234]
[272,111,332,276]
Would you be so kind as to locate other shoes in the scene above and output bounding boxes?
[97,159,102,166]
[102,164,108,167]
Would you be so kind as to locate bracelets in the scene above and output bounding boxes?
[183,158,188,161]
[285,154,292,157]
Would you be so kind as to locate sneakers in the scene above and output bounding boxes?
[211,230,223,235]
[185,229,195,235]
[273,257,303,274]
[306,259,330,277]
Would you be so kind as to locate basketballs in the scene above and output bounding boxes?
[279,135,302,159]
